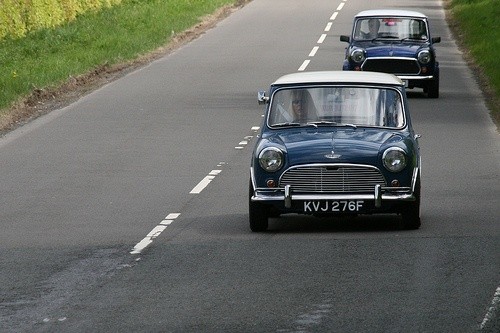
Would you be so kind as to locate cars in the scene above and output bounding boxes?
[248,70,423,232]
[339,8,443,98]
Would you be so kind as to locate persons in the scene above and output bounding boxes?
[287,89,319,122]
[363,18,382,39]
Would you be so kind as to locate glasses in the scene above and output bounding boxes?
[292,98,308,104]
